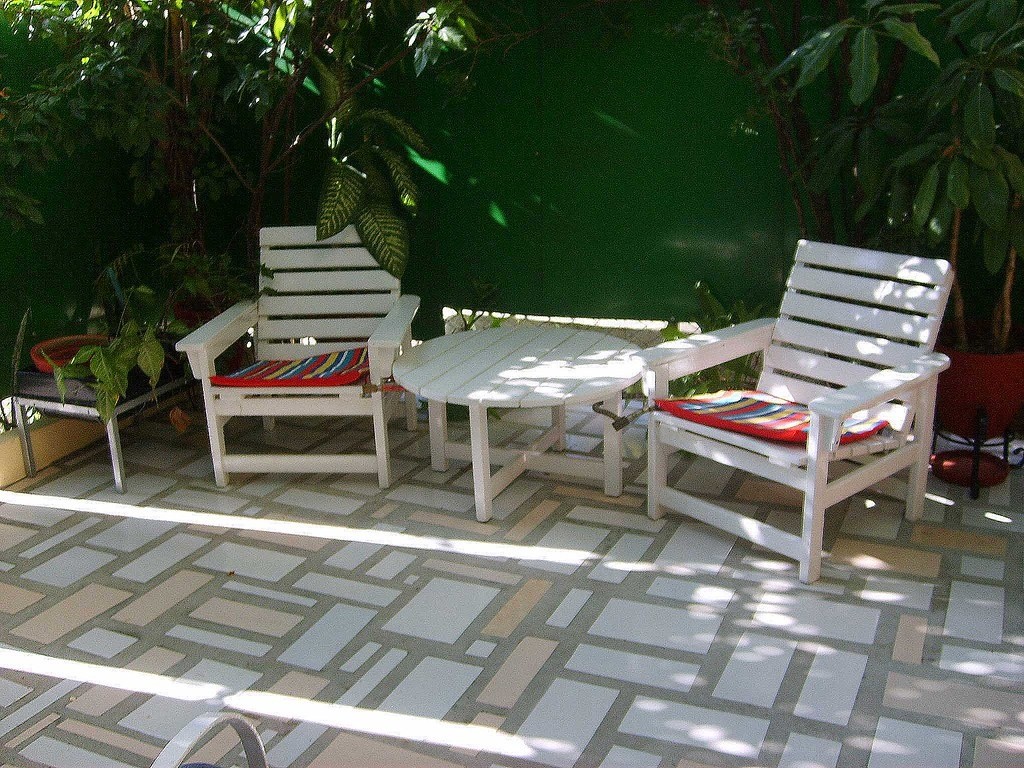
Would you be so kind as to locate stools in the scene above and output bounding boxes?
[15,371,198,493]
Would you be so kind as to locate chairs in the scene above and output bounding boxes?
[172,223,420,488]
[628,237,958,582]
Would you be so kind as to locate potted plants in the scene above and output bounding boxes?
[762,0,1024,486]
[38,241,279,422]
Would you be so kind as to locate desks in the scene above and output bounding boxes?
[392,326,647,522]
